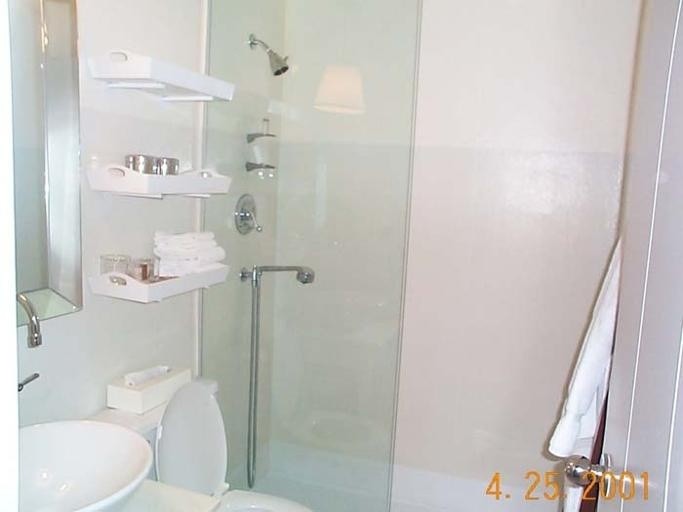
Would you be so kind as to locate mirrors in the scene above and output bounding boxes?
[10,0,85,329]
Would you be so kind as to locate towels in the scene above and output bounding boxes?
[547,236,623,464]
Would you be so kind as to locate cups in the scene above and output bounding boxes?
[124,154,179,176]
[98,253,155,285]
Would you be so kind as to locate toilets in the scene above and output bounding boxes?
[96,378,313,512]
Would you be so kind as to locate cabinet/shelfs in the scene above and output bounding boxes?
[89,48,234,303]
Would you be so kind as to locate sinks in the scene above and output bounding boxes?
[19,419,155,511]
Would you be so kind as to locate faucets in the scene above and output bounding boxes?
[16,292,43,350]
